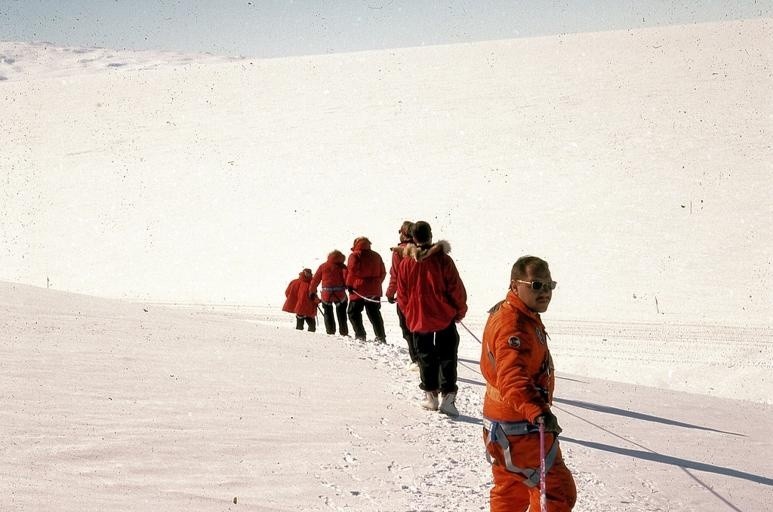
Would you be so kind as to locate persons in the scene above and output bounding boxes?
[386,221,421,370]
[282,269,321,332]
[479,255,577,512]
[346,237,387,343]
[309,250,349,338]
[397,220,468,416]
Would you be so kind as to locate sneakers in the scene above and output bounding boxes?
[355,335,366,340]
[375,337,385,342]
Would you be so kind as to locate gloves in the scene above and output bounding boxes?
[309,293,315,300]
[348,286,354,294]
[388,297,395,303]
[535,412,557,430]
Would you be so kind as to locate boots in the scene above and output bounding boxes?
[421,390,438,411]
[439,392,459,417]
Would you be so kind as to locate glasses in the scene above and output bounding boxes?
[512,279,557,290]
